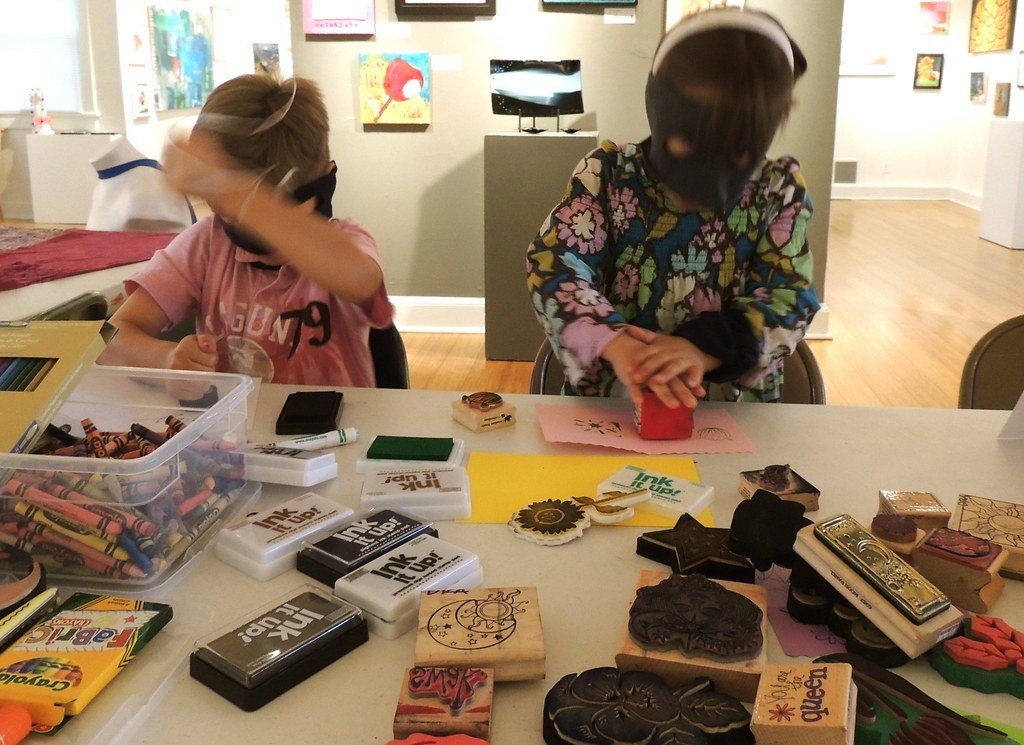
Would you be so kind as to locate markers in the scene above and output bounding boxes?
[265,427,360,451]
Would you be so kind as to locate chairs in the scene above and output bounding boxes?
[530,324,826,403]
[957,315,1024,410]
[159,315,408,388]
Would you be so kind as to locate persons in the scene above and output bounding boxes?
[97,74,396,400]
[525,6,821,408]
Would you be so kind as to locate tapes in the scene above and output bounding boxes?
[179,385,218,408]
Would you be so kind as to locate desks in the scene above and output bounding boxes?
[0,381,1023,745]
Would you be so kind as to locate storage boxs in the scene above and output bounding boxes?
[0,318,483,725]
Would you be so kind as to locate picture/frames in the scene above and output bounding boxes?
[302,1,376,36]
[913,53,947,89]
[395,0,496,16]
